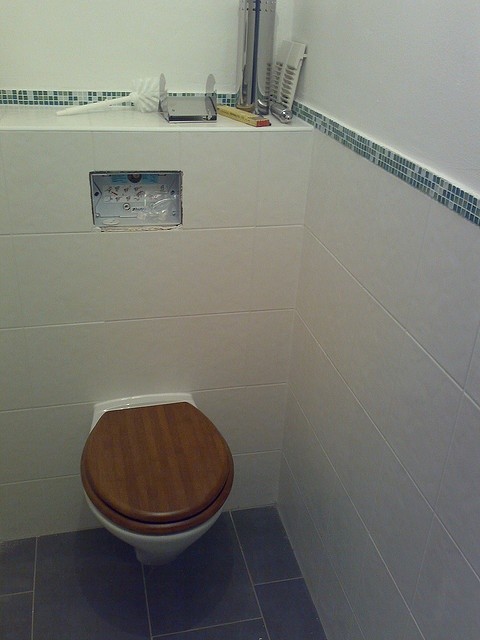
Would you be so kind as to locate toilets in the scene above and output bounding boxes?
[81,393,234,566]
